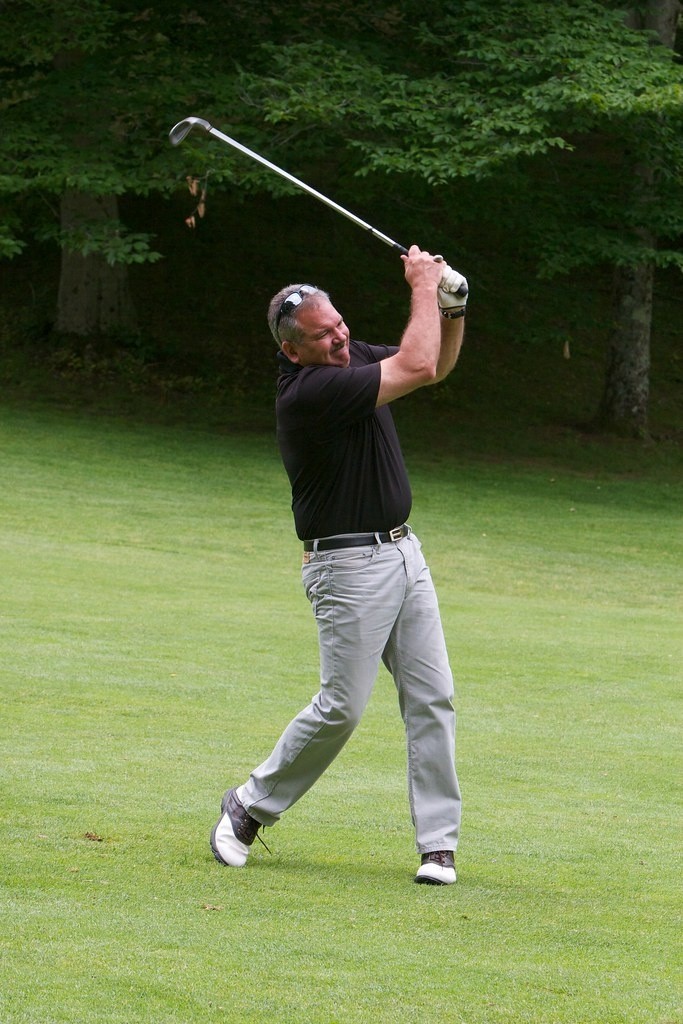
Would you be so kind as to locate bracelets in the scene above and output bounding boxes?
[440,305,467,319]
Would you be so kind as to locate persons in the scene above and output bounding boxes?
[210,245,469,885]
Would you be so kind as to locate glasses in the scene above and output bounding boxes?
[274,284,313,343]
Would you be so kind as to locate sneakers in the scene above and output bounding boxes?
[415,851,456,884]
[210,787,262,866]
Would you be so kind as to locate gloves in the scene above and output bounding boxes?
[434,254,469,307]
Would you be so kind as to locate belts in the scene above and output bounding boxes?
[304,526,407,551]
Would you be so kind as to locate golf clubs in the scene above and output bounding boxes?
[169,116,470,298]
